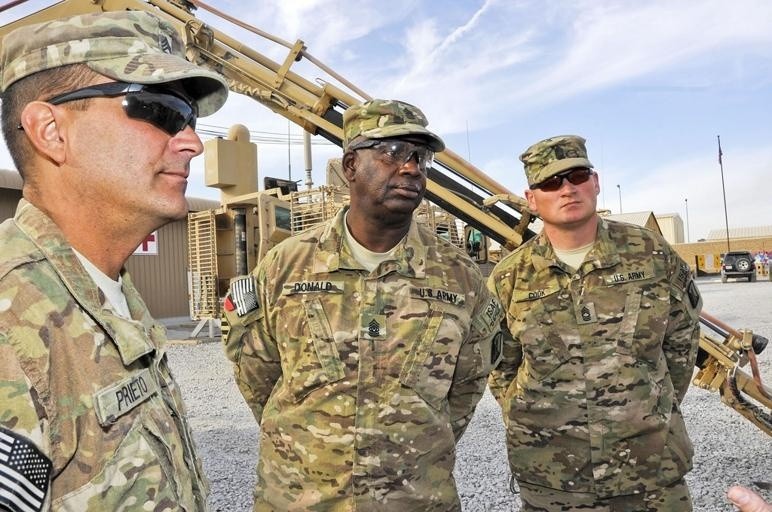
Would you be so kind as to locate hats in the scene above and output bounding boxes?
[518,133,595,191]
[342,97,445,155]
[0,9,231,120]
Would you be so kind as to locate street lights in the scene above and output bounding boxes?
[617,185,622,213]
[685,199,691,243]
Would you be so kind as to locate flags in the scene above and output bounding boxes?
[718,138,722,165]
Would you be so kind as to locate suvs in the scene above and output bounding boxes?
[720,249,757,284]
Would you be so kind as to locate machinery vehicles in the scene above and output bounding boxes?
[184,123,490,344]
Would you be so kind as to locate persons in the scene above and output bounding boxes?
[221,99,505,512]
[486,136,703,512]
[0,9,228,512]
[727,486,772,512]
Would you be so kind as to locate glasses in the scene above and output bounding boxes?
[529,167,593,193]
[350,138,436,178]
[16,80,200,137]
